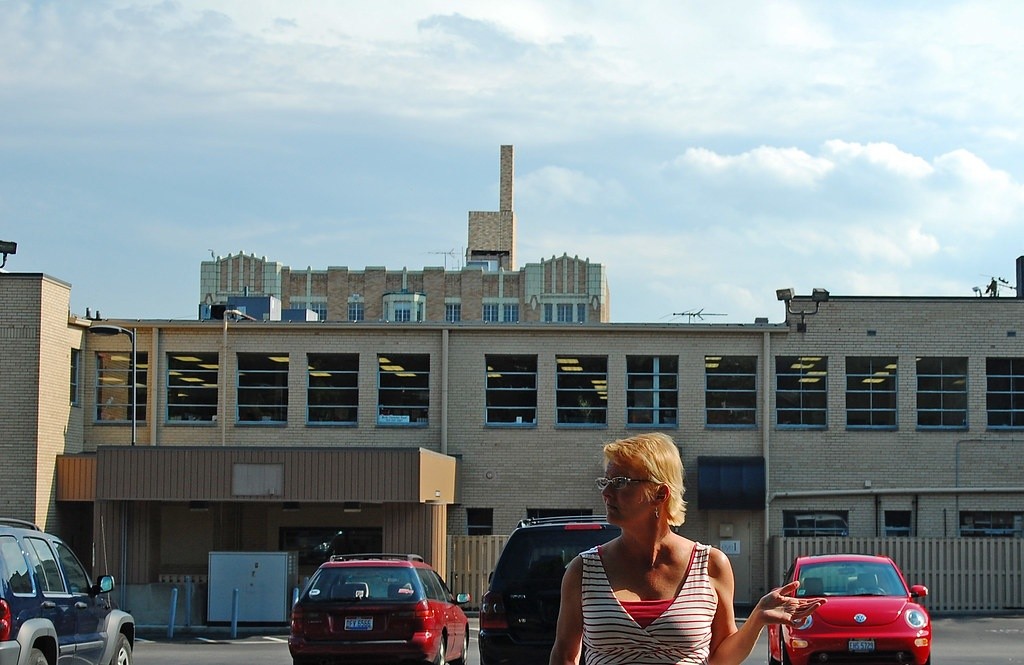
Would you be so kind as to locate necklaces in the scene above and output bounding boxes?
[619,537,663,570]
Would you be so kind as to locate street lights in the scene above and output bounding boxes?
[87,324,138,447]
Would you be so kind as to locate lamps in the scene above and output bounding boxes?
[0,240,17,268]
[776,288,829,331]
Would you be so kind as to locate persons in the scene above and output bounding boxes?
[549,433,828,665]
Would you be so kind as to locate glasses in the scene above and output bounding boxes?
[595,476,652,491]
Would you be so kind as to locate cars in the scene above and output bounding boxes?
[285,552,472,665]
[767,554,932,665]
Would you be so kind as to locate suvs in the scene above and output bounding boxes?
[0,516,136,665]
[477,514,623,665]
